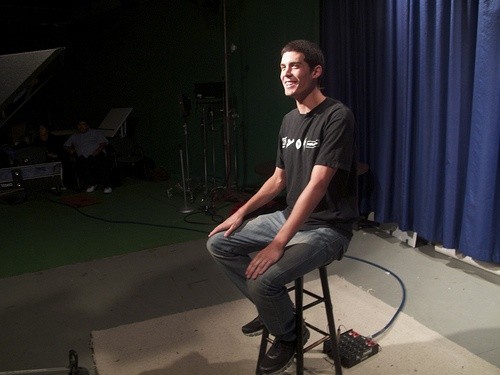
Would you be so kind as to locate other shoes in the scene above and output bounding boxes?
[104,187,112,193]
[87,184,97,192]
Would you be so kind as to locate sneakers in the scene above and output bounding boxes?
[259,324,310,374]
[242,313,268,337]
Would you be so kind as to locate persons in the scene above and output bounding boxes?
[32,124,61,163]
[207,39,358,375]
[64,119,113,194]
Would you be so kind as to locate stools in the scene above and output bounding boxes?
[255,259,343,375]
